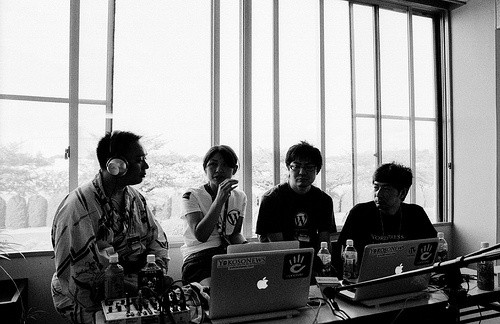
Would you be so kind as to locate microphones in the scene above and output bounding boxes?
[322,287,339,299]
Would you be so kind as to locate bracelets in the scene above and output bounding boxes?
[243,241,249,244]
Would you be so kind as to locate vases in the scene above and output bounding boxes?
[0,278,30,324]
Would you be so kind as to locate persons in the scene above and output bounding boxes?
[50,130,169,324]
[336,160,438,278]
[256,140,338,285]
[180,145,250,286]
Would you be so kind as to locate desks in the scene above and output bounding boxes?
[95,268,500,324]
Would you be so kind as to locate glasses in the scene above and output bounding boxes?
[289,164,317,171]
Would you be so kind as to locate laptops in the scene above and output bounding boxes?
[204,248,315,319]
[337,238,441,301]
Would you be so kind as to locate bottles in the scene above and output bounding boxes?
[104,253,125,300]
[141,253,163,297]
[436,231,448,263]
[342,239,358,279]
[477,241,494,291]
[316,241,332,278]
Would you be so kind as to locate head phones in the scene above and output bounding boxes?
[105,131,129,177]
